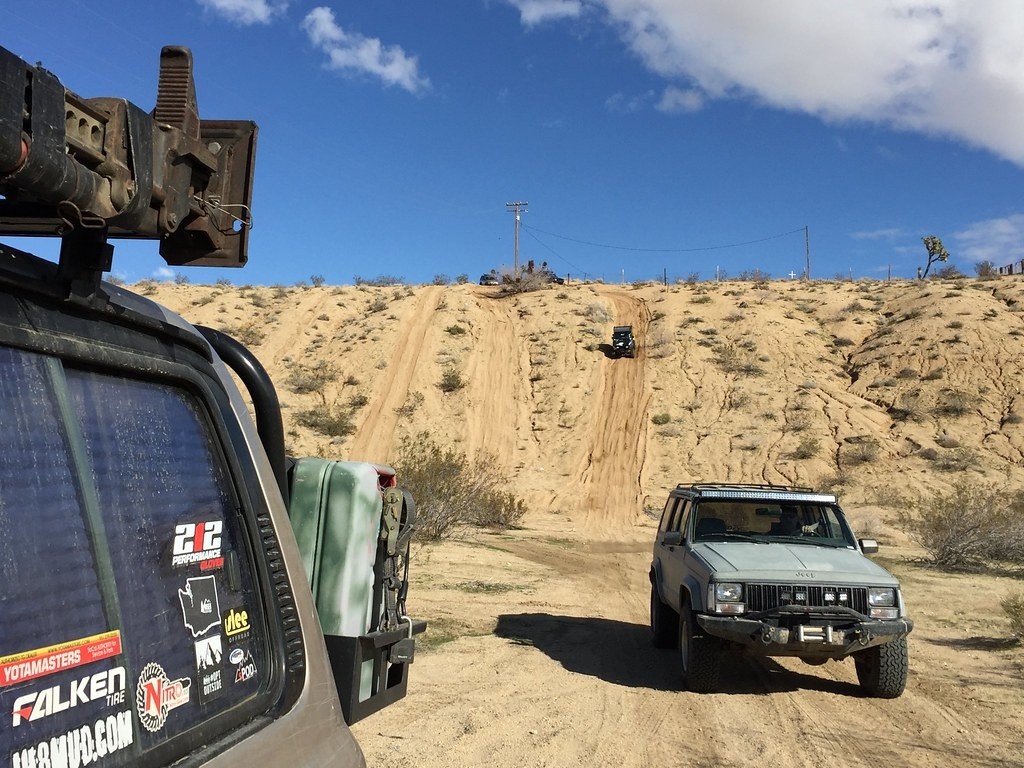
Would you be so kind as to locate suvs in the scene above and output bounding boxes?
[548,273,564,285]
[649,482,915,699]
[0,45,427,768]
[480,274,498,286]
[611,326,636,358]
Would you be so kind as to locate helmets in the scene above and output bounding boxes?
[779,505,804,531]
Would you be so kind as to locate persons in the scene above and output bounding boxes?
[918,267,922,280]
[766,506,817,537]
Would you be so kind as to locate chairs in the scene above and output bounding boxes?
[696,519,729,541]
[772,519,804,536]
